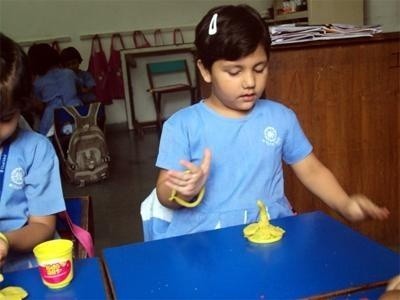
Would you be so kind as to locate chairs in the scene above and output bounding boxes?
[146,59,195,129]
[56,194,95,258]
[53,104,104,163]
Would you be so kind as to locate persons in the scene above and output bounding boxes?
[60,46,96,103]
[0,30,68,274]
[155,4,390,239]
[28,41,83,139]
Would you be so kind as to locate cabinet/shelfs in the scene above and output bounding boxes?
[273,0,365,26]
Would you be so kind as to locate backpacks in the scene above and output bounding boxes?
[53,101,113,188]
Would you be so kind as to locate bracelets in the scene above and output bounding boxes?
[169,170,207,208]
[0,232,9,244]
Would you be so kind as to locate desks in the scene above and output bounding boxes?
[1,255,111,296]
[101,210,400,297]
[192,26,400,252]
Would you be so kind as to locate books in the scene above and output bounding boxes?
[268,22,383,47]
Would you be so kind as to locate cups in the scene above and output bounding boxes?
[33,238,75,290]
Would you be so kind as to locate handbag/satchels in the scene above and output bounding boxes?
[85,35,113,105]
[107,33,128,100]
